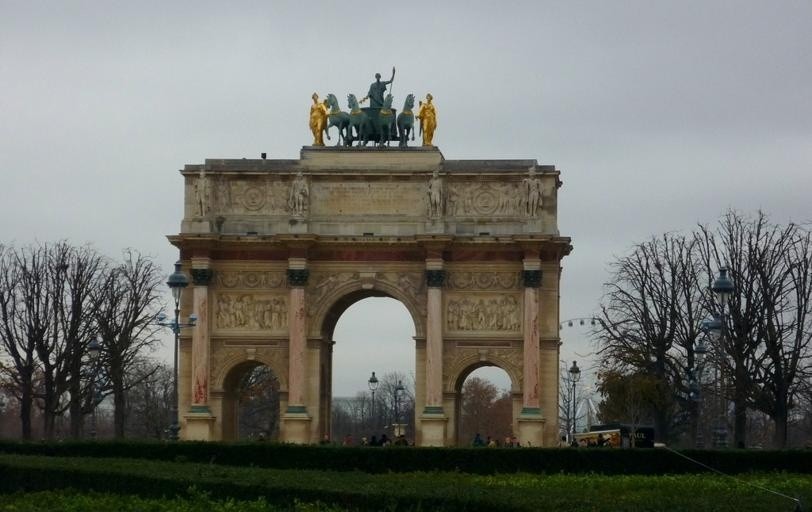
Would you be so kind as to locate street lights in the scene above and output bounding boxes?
[567,360,581,432]
[369,372,378,416]
[396,381,405,437]
[158,261,197,447]
[694,267,735,450]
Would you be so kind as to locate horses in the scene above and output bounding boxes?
[322,94,417,148]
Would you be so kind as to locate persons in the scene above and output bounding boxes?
[415,92,438,146]
[472,431,520,449]
[366,64,395,108]
[557,433,611,448]
[256,432,266,442]
[343,432,409,448]
[308,93,326,144]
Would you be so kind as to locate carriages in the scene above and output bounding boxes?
[325,66,416,147]
